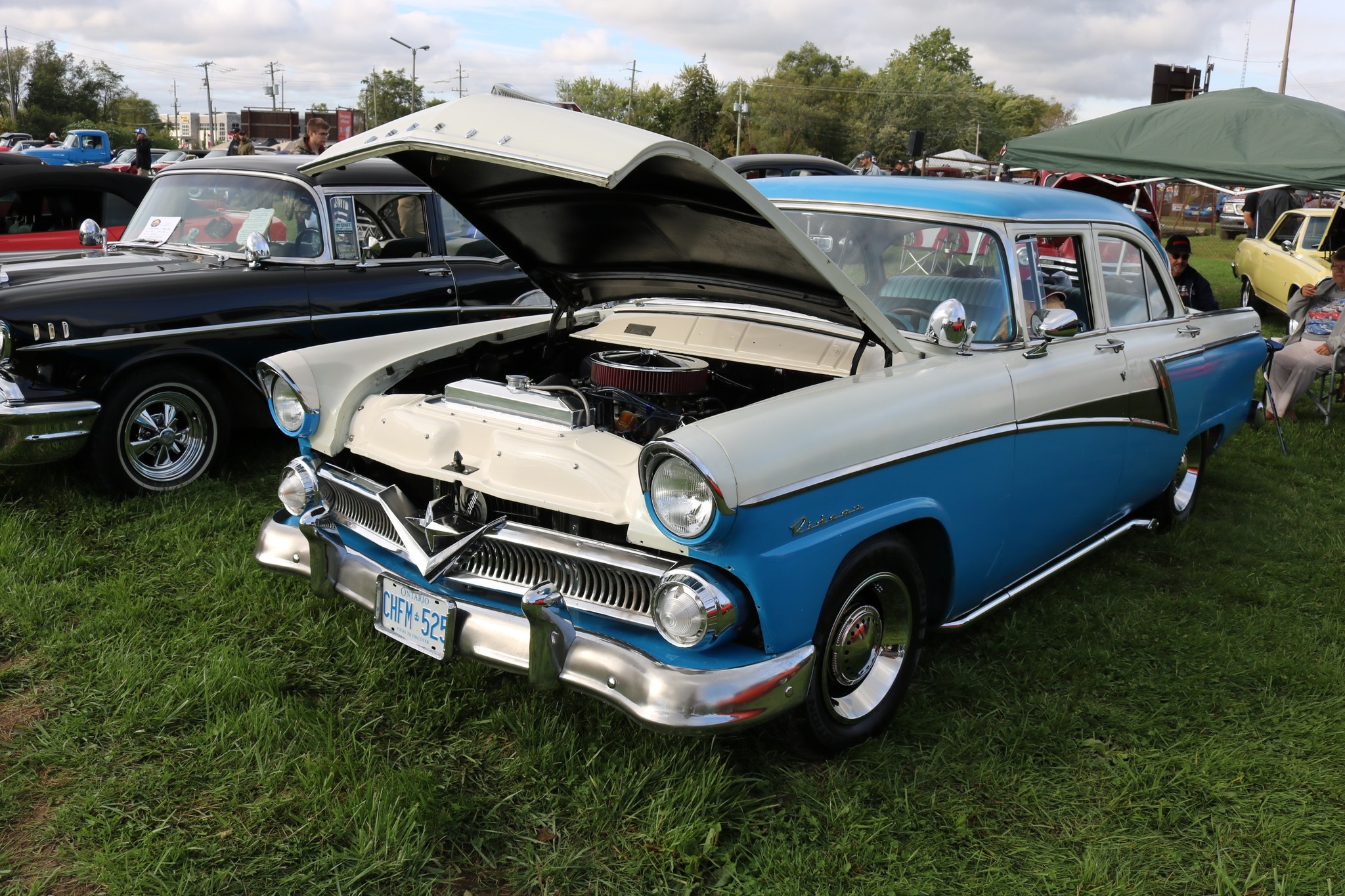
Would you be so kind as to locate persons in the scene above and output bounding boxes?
[1240,189,1305,239]
[134,127,152,177]
[1164,233,1219,313]
[397,195,425,238]
[226,127,255,156]
[891,159,955,178]
[284,118,331,232]
[1265,246,1345,424]
[44,132,58,146]
[856,151,882,176]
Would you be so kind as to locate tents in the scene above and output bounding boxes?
[970,86,1345,277]
[915,148,999,174]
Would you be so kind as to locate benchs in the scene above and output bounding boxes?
[876,275,1002,341]
[1021,279,1162,330]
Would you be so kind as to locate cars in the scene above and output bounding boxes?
[722,153,1173,286]
[0,130,556,497]
[253,95,1266,755]
[1182,186,1345,342]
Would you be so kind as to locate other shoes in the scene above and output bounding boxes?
[1265,409,1281,425]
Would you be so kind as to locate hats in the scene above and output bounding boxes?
[908,160,913,163]
[50,132,58,138]
[896,160,904,163]
[238,130,246,134]
[134,127,146,135]
[1167,234,1192,255]
[857,151,872,159]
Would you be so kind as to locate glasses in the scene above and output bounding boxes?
[1331,262,1345,270]
[899,162,903,165]
[1168,249,1190,260]
[239,133,245,135]
[318,132,329,137]
[230,128,240,132]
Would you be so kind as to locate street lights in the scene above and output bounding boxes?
[390,37,430,114]
[717,112,741,156]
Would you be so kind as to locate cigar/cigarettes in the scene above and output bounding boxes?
[1307,288,1314,290]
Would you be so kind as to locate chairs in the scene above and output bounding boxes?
[1259,334,1345,425]
[899,226,996,278]
